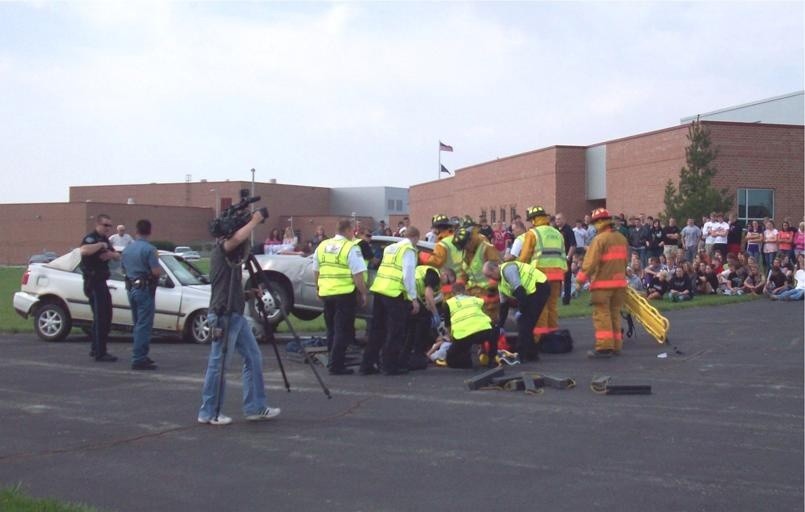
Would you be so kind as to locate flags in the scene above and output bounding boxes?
[441,163,452,176]
[440,143,453,152]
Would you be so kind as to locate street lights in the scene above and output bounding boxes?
[351,210,358,233]
[288,215,293,229]
[209,187,220,222]
[249,168,256,248]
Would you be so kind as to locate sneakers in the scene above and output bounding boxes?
[132,356,158,370]
[245,405,281,422]
[669,287,793,302]
[89,349,118,362]
[586,348,622,360]
[196,410,233,426]
[328,352,542,376]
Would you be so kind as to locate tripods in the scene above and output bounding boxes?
[214,239,333,422]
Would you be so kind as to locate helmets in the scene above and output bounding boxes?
[525,204,550,221]
[586,207,613,225]
[451,227,471,251]
[430,214,475,228]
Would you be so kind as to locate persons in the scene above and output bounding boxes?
[314,206,628,377]
[195,212,282,427]
[78,214,122,362]
[629,202,805,301]
[107,224,135,248]
[264,205,629,305]
[120,218,163,372]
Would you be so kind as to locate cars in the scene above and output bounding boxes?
[10,244,262,345]
[173,243,201,264]
[26,251,60,268]
[241,235,438,327]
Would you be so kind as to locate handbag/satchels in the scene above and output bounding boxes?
[539,327,574,354]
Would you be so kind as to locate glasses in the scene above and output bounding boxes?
[100,223,113,228]
